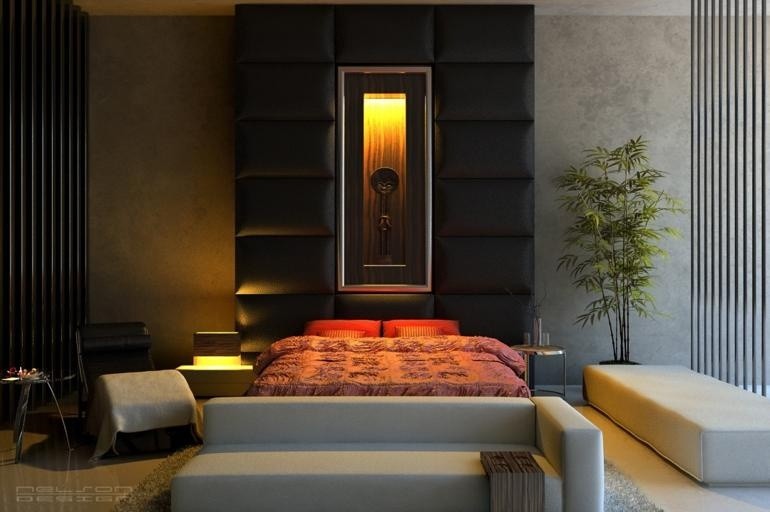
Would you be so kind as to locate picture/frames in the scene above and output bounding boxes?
[338,66,433,293]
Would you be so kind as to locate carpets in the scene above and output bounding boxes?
[114,444,664,512]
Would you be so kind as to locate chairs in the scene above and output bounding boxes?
[75,322,204,466]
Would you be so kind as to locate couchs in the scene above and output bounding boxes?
[170,395,605,512]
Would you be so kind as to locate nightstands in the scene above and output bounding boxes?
[176,365,254,397]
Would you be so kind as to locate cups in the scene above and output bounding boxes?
[543,333,549,345]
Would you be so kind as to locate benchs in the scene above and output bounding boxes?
[583,364,770,488]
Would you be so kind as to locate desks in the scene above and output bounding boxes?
[0,369,75,464]
[510,345,566,397]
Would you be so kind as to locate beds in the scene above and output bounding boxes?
[244,335,531,397]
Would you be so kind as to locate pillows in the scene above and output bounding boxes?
[302,320,461,338]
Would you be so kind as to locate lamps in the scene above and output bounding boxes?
[192,331,241,368]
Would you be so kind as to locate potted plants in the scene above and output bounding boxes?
[548,135,689,364]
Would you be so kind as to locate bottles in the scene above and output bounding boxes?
[523,332,530,344]
